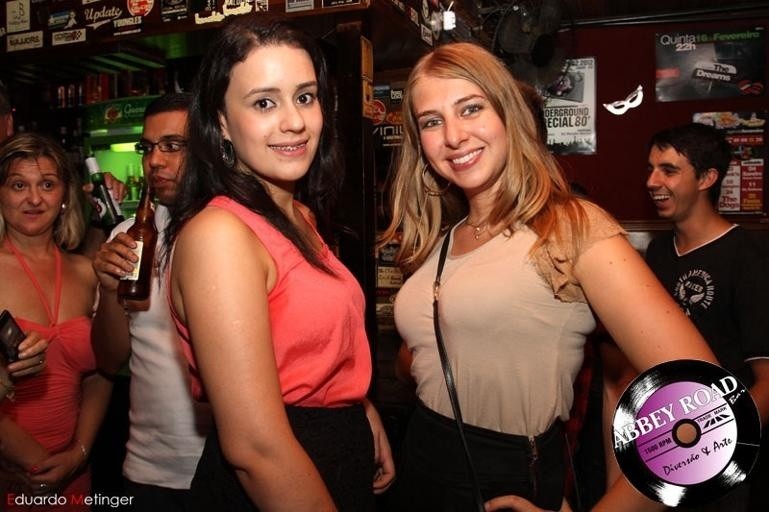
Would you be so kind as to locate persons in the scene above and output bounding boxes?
[394,42,721,512]
[601,120,768,490]
[0,10,395,512]
[515,77,550,143]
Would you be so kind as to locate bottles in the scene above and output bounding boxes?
[59,127,158,301]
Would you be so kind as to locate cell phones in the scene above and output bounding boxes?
[0,310,27,364]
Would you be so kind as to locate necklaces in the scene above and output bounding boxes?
[463,220,493,241]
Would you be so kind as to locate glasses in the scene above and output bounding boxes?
[135,140,187,155]
